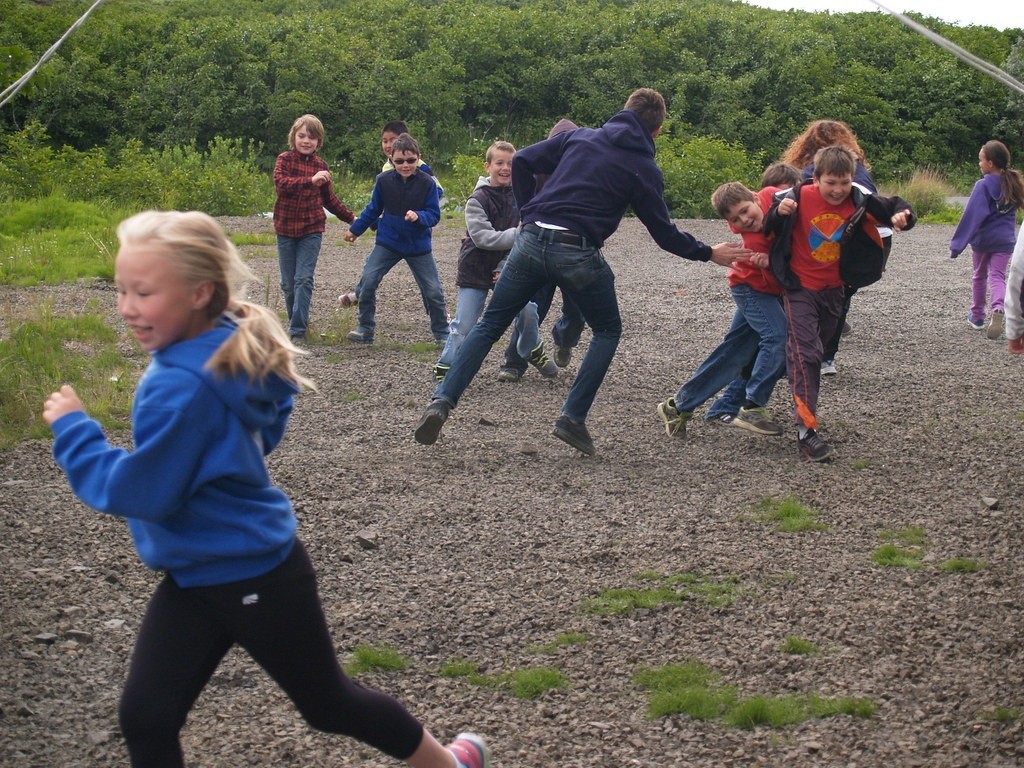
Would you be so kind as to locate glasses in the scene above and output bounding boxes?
[392,159,419,164]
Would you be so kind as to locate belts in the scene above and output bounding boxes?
[524,223,595,247]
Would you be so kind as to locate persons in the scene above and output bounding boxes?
[271,113,357,347]
[436,142,560,380]
[703,162,803,426]
[498,115,586,385]
[1002,217,1024,362]
[784,119,882,375]
[337,121,443,308]
[343,133,453,346]
[415,88,758,457]
[760,144,918,462]
[949,138,1024,340]
[40,205,491,768]
[657,183,783,442]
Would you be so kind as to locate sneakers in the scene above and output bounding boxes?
[656,397,693,439]
[434,364,450,383]
[796,425,831,462]
[713,412,735,426]
[733,406,784,435]
[553,334,572,368]
[498,366,518,383]
[525,341,559,378]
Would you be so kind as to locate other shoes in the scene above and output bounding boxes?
[820,361,836,377]
[291,333,304,345]
[553,411,597,456]
[988,310,1005,339]
[415,397,450,444]
[338,292,358,308]
[840,321,854,336]
[967,312,985,328]
[347,329,376,344]
[446,733,488,768]
